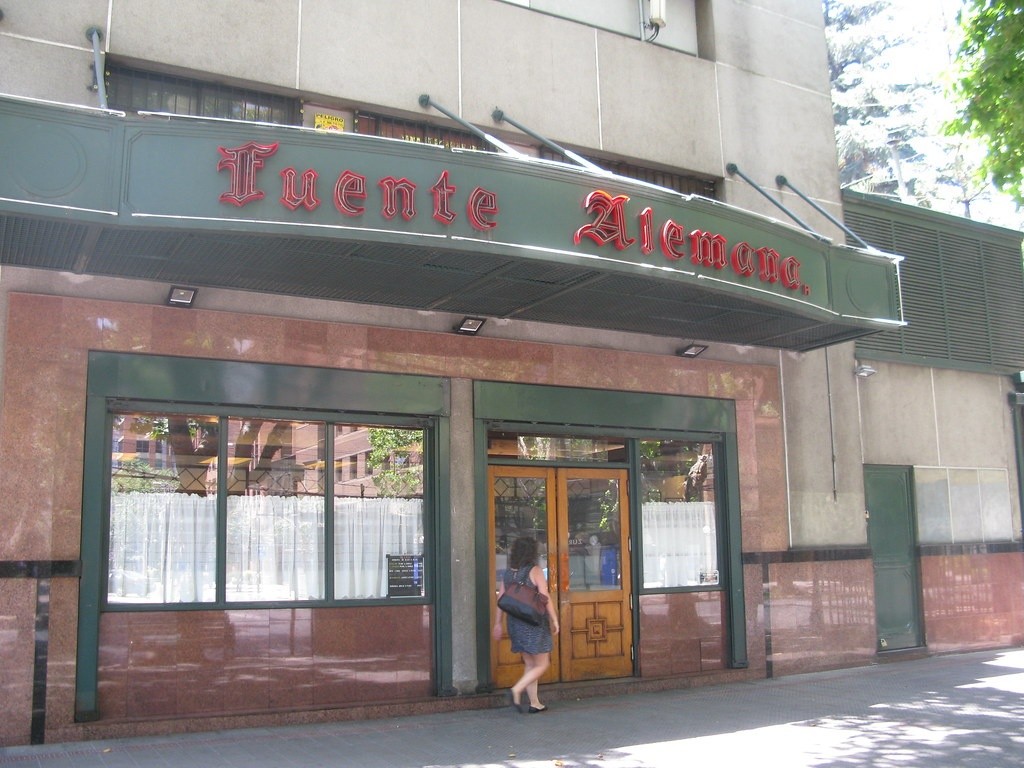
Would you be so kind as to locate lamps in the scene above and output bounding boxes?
[167,284,200,309]
[675,344,709,357]
[452,316,487,336]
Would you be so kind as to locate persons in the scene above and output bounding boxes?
[494,537,559,714]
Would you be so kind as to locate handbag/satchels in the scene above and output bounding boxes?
[498,564,548,627]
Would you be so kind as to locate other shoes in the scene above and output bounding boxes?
[507,688,523,714]
[528,705,547,713]
